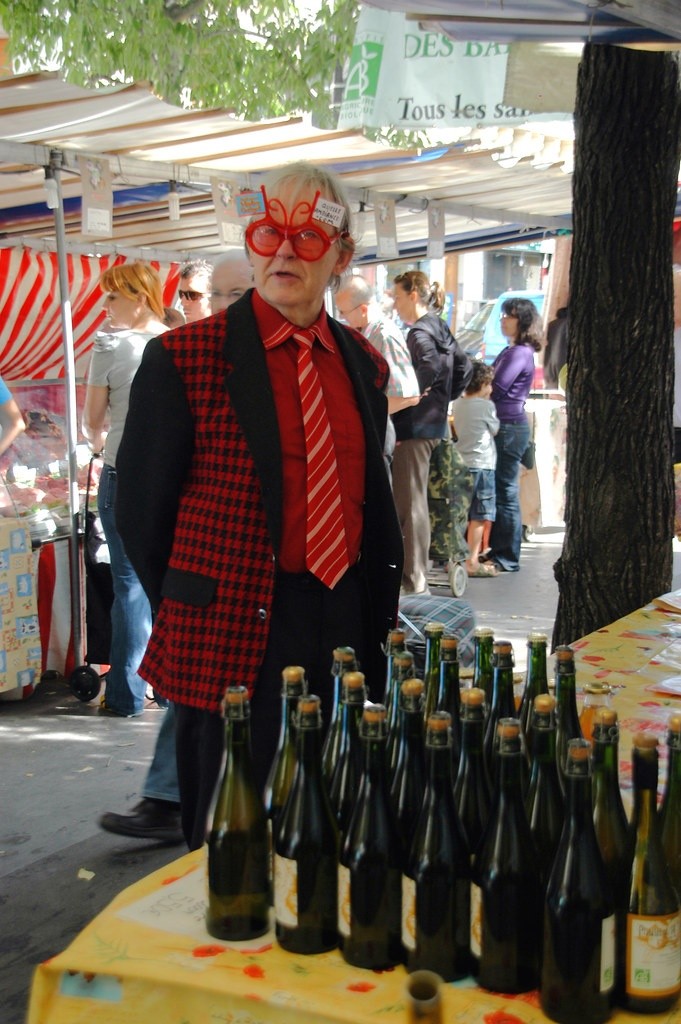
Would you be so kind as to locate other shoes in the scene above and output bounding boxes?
[469,564,499,577]
[98,706,133,718]
[401,588,431,596]
[99,800,183,843]
[478,553,508,572]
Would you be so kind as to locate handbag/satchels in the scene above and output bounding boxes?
[520,441,536,470]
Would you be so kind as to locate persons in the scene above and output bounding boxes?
[453,360,500,580]
[84,247,253,842]
[542,299,569,391]
[332,276,418,507]
[0,377,25,463]
[387,270,471,594]
[489,296,539,575]
[114,160,406,853]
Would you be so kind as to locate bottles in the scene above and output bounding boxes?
[203,621,681,1024]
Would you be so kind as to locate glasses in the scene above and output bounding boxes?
[335,302,364,315]
[209,288,241,302]
[499,313,517,319]
[178,289,206,301]
[246,183,348,262]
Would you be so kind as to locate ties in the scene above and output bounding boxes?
[292,335,351,589]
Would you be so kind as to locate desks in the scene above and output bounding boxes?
[26,603,681,1024]
